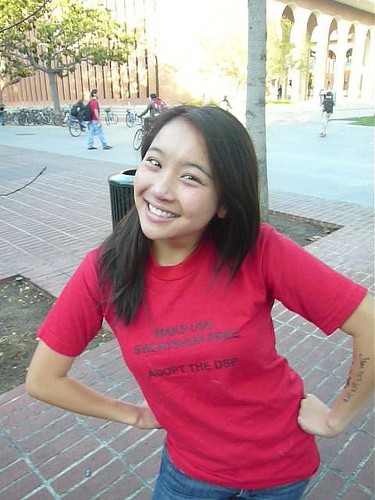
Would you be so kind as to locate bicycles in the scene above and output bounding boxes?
[105,105,119,126]
[133,115,154,150]
[125,105,144,128]
[5,104,102,137]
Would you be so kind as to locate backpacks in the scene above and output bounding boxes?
[152,97,167,111]
[325,102,333,112]
[71,105,81,116]
[80,107,92,120]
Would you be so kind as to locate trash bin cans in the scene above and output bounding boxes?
[105,165,136,232]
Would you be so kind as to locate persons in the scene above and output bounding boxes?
[73,88,336,149]
[25,102,375,500]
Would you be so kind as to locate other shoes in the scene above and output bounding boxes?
[103,146,112,149]
[88,146,97,149]
[319,132,326,137]
